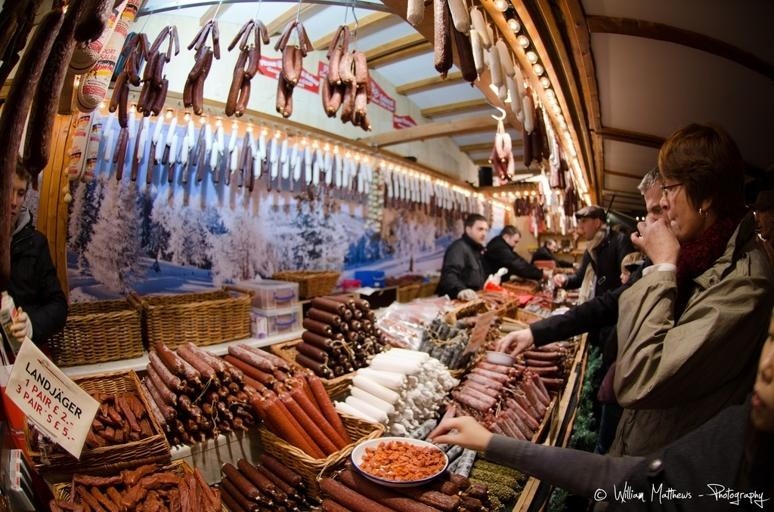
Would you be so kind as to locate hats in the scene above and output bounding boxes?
[575,205,605,220]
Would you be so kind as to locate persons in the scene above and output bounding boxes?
[426,299,774,511]
[493,163,674,456]
[610,223,632,238]
[586,250,646,434]
[3,152,69,363]
[529,238,556,267]
[483,225,554,281]
[552,238,576,267]
[747,191,773,268]
[431,212,491,303]
[549,205,636,366]
[605,120,774,460]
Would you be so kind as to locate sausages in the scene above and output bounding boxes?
[1,2,139,277]
[0,285,579,512]
[106,2,595,240]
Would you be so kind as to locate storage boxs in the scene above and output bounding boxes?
[238,278,303,339]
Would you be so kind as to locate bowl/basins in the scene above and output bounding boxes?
[485,350,517,368]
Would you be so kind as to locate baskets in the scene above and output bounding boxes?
[384,273,441,303]
[21,368,173,476]
[53,457,234,512]
[270,328,409,398]
[255,410,387,507]
[450,277,545,327]
[130,284,256,354]
[43,297,145,367]
[446,349,484,379]
[271,268,341,299]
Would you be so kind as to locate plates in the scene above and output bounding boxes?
[350,436,449,489]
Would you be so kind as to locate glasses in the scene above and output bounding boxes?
[659,182,683,194]
